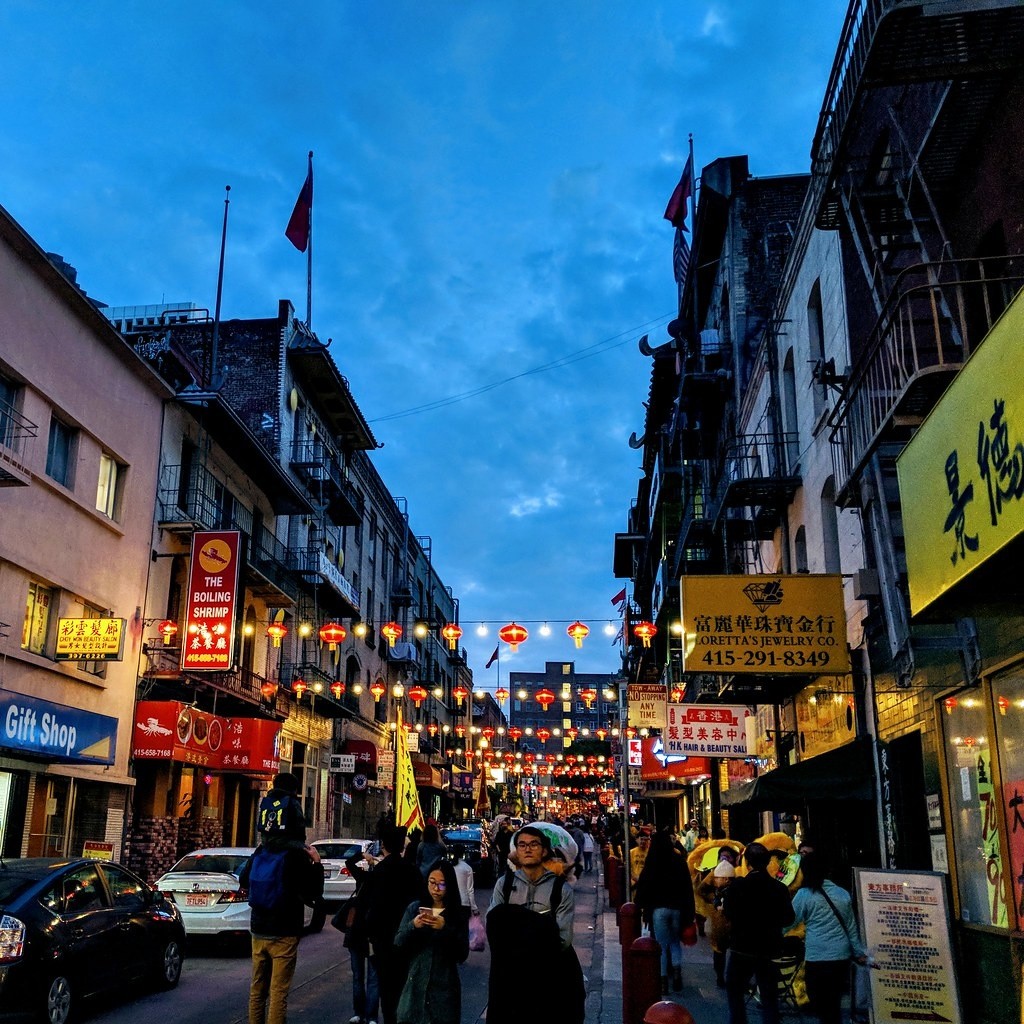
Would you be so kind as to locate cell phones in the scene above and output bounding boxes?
[419,907,433,918]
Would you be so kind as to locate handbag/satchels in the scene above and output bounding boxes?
[848,946,880,969]
[641,922,651,938]
[330,874,366,933]
[467,913,486,950]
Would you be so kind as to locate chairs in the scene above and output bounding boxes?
[66,880,96,909]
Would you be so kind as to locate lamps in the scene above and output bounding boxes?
[764,729,797,742]
[815,688,855,704]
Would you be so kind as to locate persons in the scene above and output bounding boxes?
[554,811,624,873]
[492,821,513,877]
[346,824,481,1024]
[239,772,325,1024]
[630,818,867,1024]
[485,826,575,949]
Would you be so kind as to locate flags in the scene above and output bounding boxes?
[284,175,311,253]
[611,588,626,606]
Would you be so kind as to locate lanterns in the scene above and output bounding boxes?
[446,749,614,778]
[626,727,636,739]
[443,624,462,649]
[633,621,658,647]
[293,680,598,711]
[499,624,528,651]
[404,723,608,741]
[267,623,287,648]
[526,786,614,795]
[320,622,346,651]
[158,620,178,644]
[670,688,684,703]
[382,621,403,648]
[568,621,589,648]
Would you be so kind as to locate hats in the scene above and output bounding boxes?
[713,860,736,878]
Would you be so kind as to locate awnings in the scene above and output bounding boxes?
[410,760,441,790]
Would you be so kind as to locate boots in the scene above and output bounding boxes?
[673,966,683,993]
[660,975,670,995]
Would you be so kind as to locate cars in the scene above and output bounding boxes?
[152,846,327,959]
[309,837,385,901]
[438,827,500,889]
[0,858,187,1024]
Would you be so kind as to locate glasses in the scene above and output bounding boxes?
[427,879,447,891]
[517,841,543,851]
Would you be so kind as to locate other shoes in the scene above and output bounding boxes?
[716,979,725,989]
[349,1015,361,1023]
[369,1021,376,1024]
